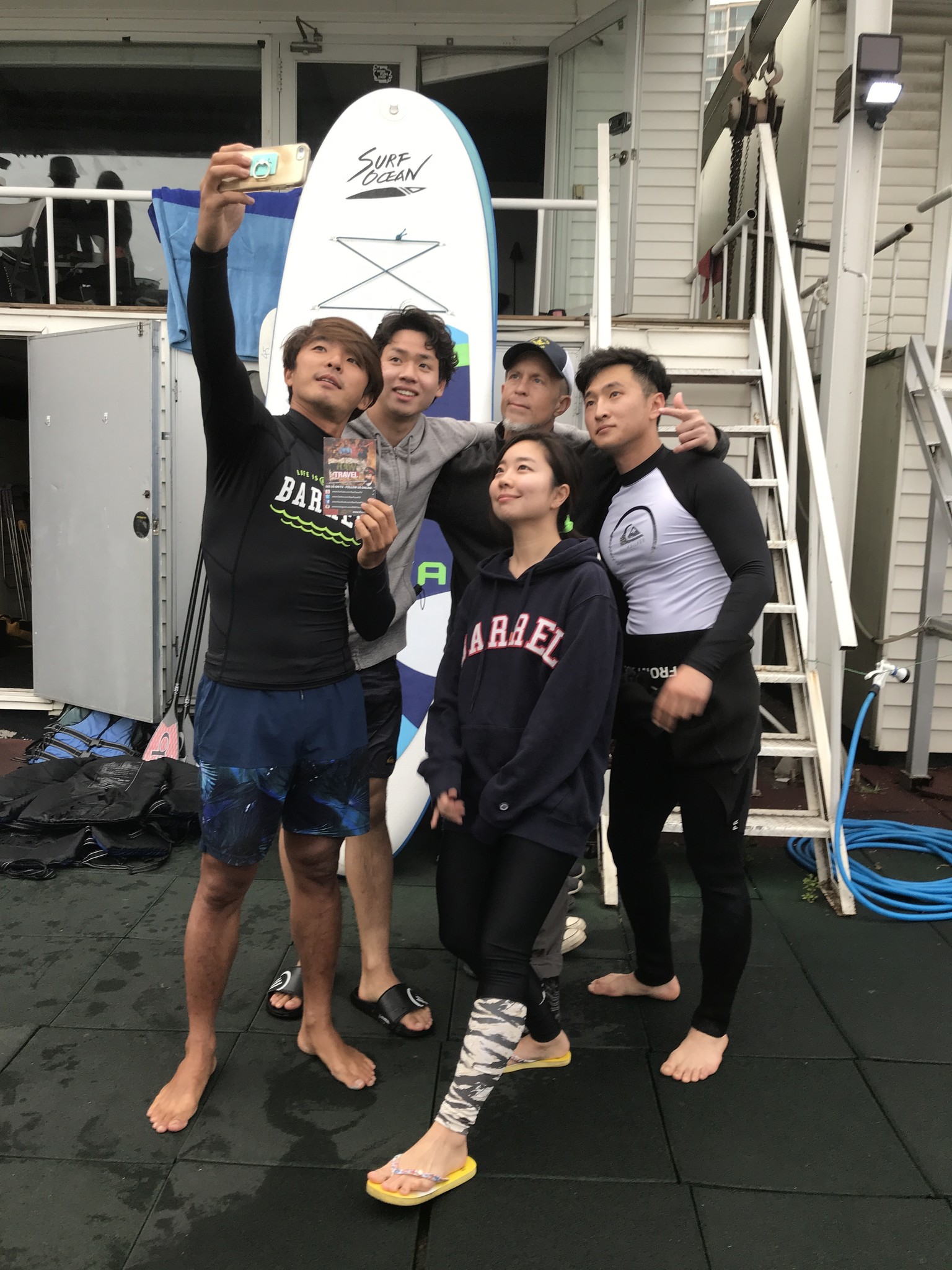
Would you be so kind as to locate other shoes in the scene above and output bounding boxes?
[566,915,587,932]
[571,863,586,876]
[566,879,583,894]
[559,928,587,954]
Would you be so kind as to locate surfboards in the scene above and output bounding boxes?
[259,81,507,883]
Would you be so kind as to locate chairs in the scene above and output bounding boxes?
[0,198,47,304]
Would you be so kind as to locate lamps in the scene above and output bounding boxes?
[856,33,903,133]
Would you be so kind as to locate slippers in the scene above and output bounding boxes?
[266,967,305,1019]
[502,1046,573,1074]
[351,982,432,1038]
[367,1152,477,1207]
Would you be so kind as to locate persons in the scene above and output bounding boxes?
[263,306,778,1204]
[22,159,133,314]
[145,141,400,1134]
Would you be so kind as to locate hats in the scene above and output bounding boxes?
[502,337,568,379]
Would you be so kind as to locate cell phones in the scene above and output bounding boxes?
[217,142,312,192]
